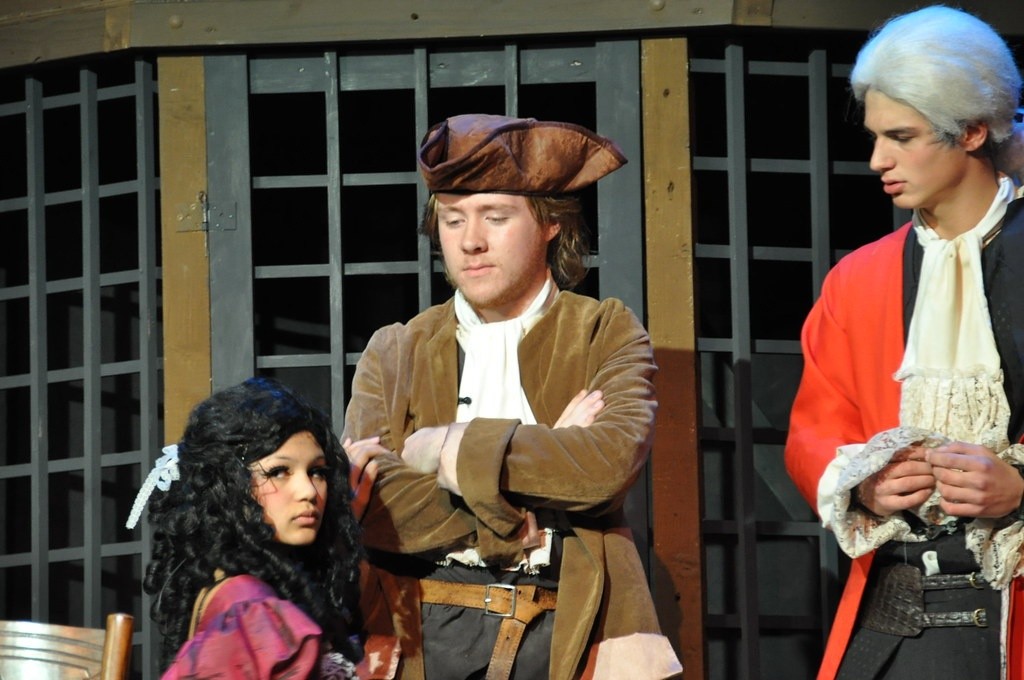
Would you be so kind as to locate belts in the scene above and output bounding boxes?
[419,577,556,680]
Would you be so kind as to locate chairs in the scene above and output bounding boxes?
[0,614,134,680]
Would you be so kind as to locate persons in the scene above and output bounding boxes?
[339,114,685,679]
[142,374,393,679]
[783,5,1024,680]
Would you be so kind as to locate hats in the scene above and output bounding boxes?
[419,112,629,196]
